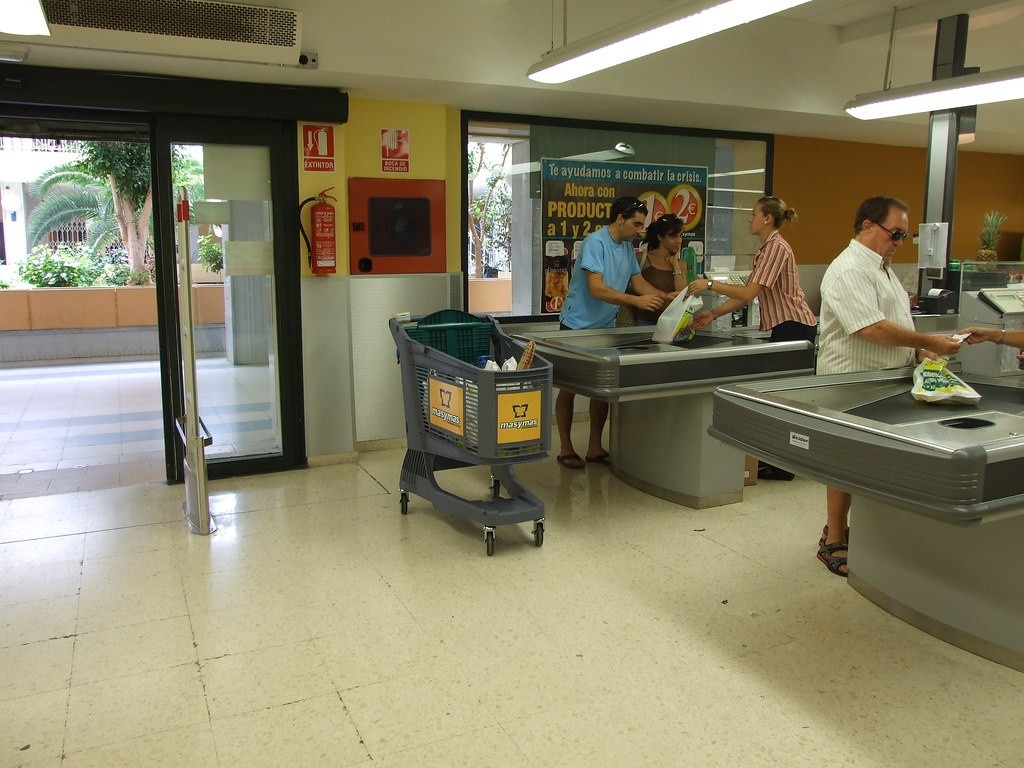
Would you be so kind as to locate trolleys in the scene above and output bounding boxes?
[388,308,553,556]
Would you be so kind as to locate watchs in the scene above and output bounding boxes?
[707,280,713,290]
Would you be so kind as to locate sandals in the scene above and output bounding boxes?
[819,526,849,546]
[817,543,848,577]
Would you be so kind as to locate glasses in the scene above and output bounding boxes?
[870,220,907,242]
[620,202,645,215]
[659,215,677,224]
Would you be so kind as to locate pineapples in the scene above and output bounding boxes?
[976,210,1008,272]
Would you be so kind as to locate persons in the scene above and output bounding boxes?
[816,196,962,577]
[957,327,1024,360]
[555,197,688,469]
[636,212,688,326]
[689,197,817,481]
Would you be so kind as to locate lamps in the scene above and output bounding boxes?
[525,0,1024,121]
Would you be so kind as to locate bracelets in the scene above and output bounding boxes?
[673,269,681,276]
[915,348,924,364]
[710,309,716,320]
[996,330,1006,345]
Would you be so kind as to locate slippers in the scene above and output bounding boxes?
[557,454,585,469]
[586,451,610,465]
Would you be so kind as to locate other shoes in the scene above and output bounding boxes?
[758,466,794,481]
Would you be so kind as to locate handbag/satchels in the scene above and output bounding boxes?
[911,355,984,405]
[652,283,703,343]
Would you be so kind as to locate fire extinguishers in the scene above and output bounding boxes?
[300,186,338,275]
